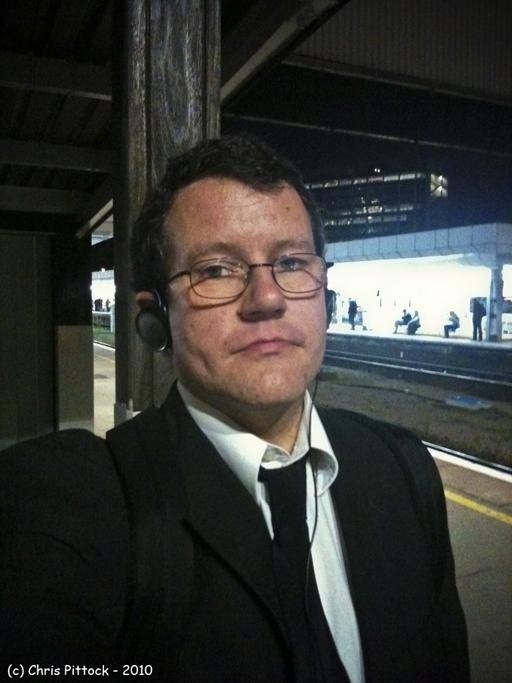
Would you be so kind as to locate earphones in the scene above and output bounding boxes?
[323,289,337,329]
[133,286,171,353]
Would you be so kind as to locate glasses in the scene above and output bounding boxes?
[169,253,334,298]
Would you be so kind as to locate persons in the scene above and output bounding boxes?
[392,308,412,334]
[1,139,470,682]
[443,309,460,339]
[348,295,358,330]
[408,311,421,336]
[469,296,485,342]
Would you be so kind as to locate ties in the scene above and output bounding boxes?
[258,448,350,682]
